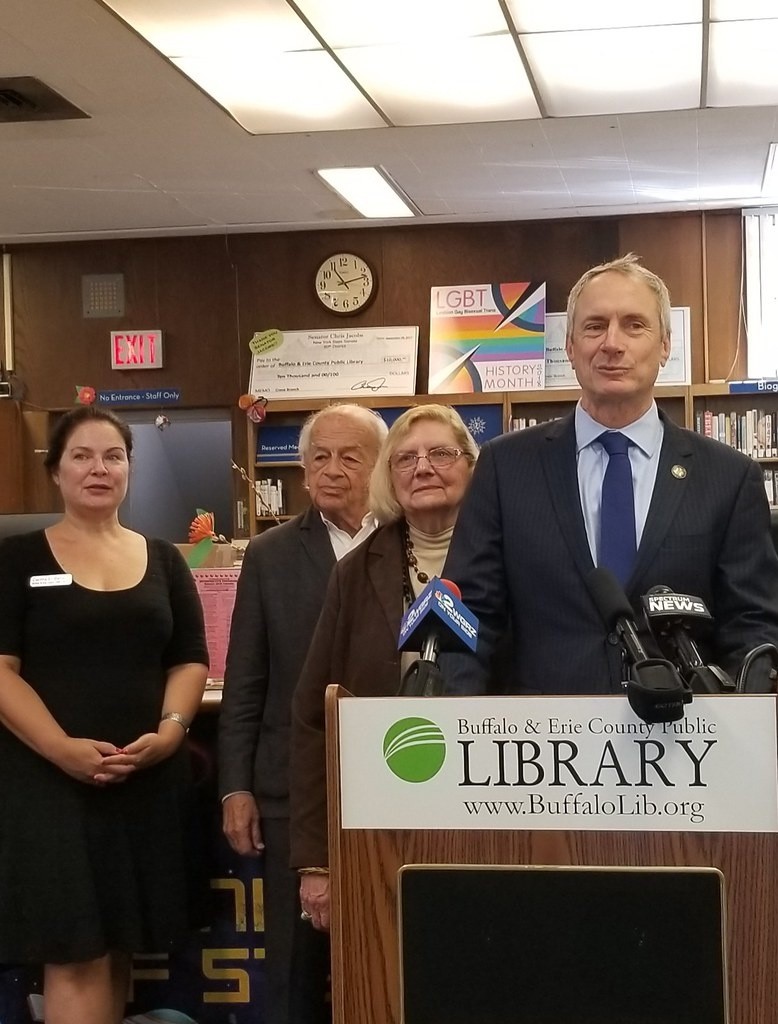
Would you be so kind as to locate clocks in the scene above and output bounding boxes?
[313,252,378,318]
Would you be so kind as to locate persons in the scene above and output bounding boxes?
[434,257,778,696]
[215,402,390,1024]
[0,406,209,1024]
[289,403,480,935]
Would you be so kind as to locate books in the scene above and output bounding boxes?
[696,410,778,506]
[512,417,562,432]
[254,478,287,516]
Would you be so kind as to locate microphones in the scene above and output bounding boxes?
[580,568,738,724]
[396,576,479,697]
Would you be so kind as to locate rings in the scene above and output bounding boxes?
[300,910,311,920]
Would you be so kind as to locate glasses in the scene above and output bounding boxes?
[390,447,470,472]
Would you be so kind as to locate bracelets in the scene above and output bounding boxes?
[159,712,189,746]
[297,867,329,876]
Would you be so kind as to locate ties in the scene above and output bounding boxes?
[597,430,638,585]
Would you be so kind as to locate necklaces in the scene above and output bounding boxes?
[402,526,430,585]
[400,565,412,607]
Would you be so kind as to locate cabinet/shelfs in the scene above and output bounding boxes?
[244,380,778,542]
